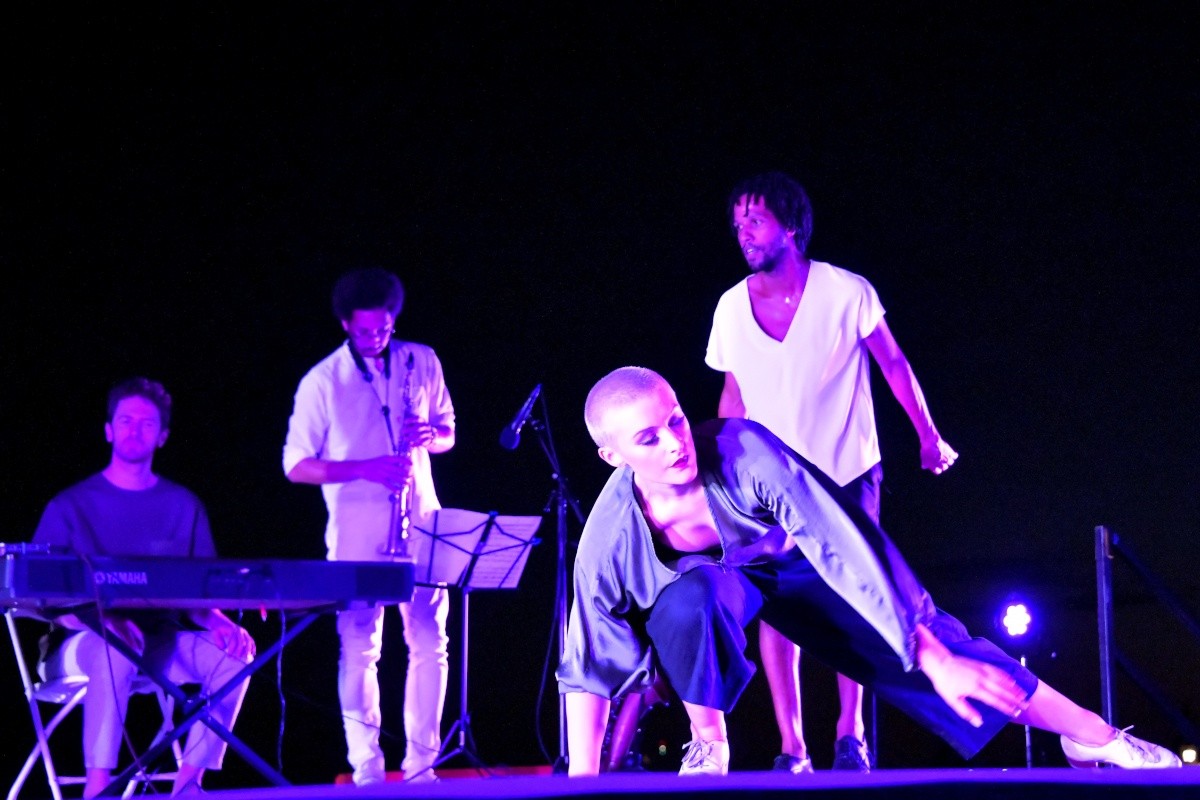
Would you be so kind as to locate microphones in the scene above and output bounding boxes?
[500,386,540,449]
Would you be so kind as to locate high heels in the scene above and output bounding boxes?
[677,736,730,776]
[1060,724,1183,769]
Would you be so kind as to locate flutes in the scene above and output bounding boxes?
[371,345,417,560]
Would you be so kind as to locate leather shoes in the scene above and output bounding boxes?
[828,735,875,776]
[770,753,815,776]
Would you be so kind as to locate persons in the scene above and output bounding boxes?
[557,367,1184,783]
[21,379,257,799]
[706,174,958,768]
[283,272,457,785]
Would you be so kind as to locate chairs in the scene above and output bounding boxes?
[7,606,184,800]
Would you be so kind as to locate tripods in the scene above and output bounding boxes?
[394,511,536,783]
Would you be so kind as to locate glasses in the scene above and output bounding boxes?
[349,325,396,338]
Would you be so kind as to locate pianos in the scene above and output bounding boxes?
[0,540,421,799]
